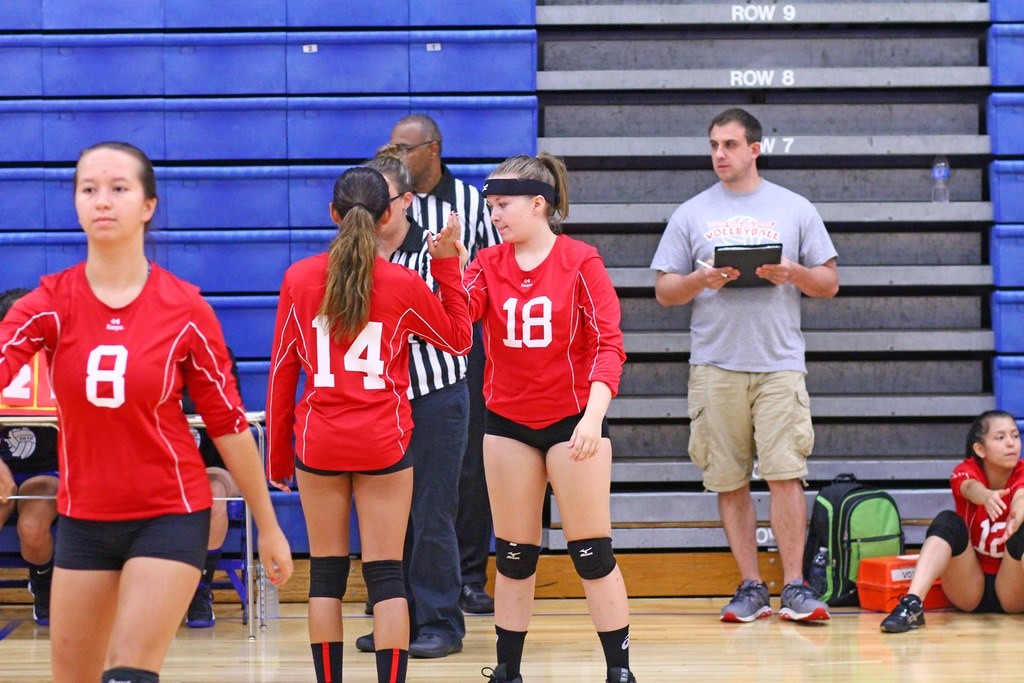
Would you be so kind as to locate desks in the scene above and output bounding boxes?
[0,412,271,644]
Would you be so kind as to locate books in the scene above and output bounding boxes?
[714,244,783,288]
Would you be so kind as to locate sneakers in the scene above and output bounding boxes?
[720,578,772,623]
[879,594,926,632]
[459,582,495,614]
[409,633,463,657]
[27,579,51,625]
[777,578,831,621]
[184,581,215,628]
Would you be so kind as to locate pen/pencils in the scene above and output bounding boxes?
[696,258,729,278]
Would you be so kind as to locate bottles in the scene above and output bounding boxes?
[931,155,950,202]
[256,559,279,619]
[809,547,828,599]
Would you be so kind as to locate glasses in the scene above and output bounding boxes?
[403,141,431,155]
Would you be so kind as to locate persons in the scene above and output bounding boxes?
[650,108,840,625]
[437,149,636,683]
[881,410,1024,634]
[356,113,505,659]
[0,142,294,683]
[0,288,59,626]
[179,346,243,628]
[265,167,472,683]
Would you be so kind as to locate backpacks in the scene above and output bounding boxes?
[800,472,906,606]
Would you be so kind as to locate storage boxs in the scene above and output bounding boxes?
[856,555,951,613]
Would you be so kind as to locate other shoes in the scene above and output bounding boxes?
[605,667,637,683]
[481,663,523,683]
[356,632,375,651]
